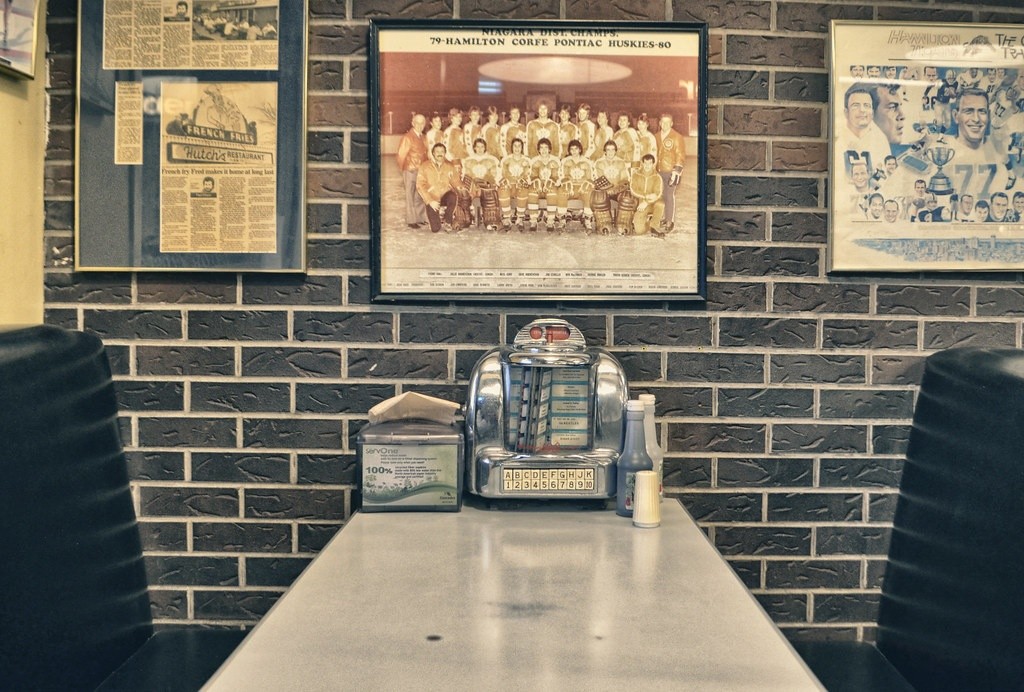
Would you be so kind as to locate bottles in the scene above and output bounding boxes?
[631,393,663,507]
[616,400,654,518]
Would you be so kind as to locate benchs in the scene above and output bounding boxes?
[0,324,251,692]
[786,346,1024,692]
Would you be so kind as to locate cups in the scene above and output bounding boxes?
[633,470,660,528]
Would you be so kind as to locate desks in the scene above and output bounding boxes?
[199,497,828,692]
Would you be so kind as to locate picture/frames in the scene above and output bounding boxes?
[368,16,708,303]
[828,19,1024,274]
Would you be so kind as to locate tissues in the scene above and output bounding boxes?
[353,390,469,515]
